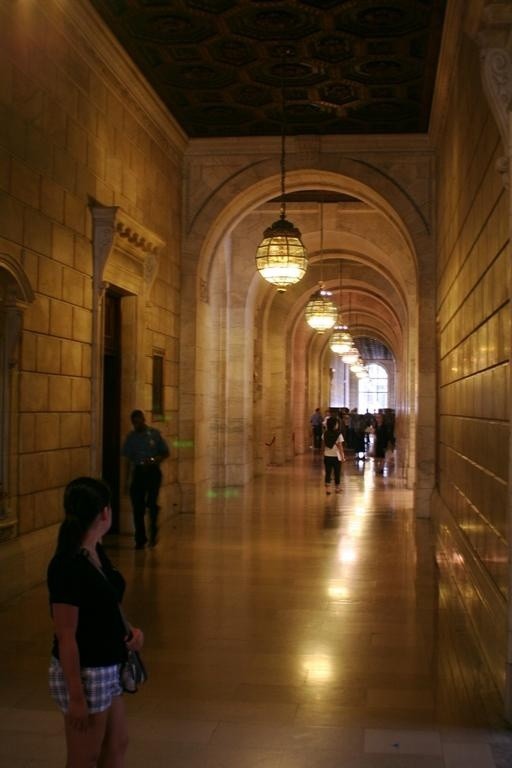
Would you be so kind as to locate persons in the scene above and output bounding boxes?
[118,410,169,552]
[47,476,144,767]
[309,407,395,494]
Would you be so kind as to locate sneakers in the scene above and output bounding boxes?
[326,487,342,494]
[134,534,158,549]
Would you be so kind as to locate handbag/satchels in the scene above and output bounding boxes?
[119,641,147,694]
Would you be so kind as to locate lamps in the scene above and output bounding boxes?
[306,204,373,387]
[254,126,310,295]
